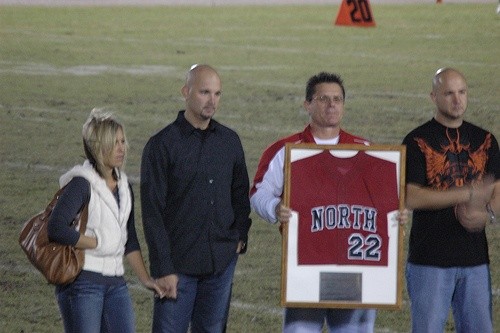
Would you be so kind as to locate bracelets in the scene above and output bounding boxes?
[466,183,475,203]
[485,201,496,225]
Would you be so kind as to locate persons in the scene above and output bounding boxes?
[46,109,167,333]
[400,67,500,333]
[251,71,411,333]
[139,63,252,333]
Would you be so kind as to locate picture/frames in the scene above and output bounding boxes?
[278,142,408,310]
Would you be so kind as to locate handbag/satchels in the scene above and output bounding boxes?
[19,177,90,286]
